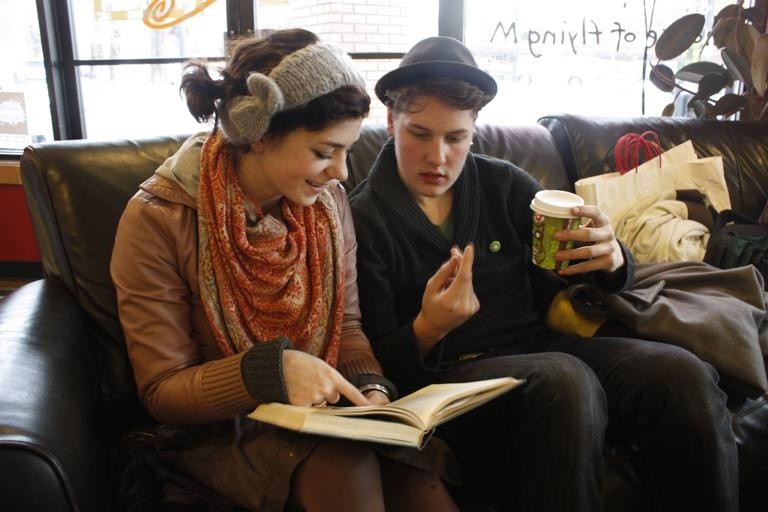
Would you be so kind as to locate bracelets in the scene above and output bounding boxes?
[359,383,390,397]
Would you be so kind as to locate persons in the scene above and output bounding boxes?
[348,37,741,512]
[110,28,463,511]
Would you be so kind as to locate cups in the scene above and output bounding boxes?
[527,189,585,272]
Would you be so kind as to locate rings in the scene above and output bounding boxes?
[316,400,328,408]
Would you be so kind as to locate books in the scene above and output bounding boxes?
[246,372,528,453]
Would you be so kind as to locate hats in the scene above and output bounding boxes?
[375,36,497,107]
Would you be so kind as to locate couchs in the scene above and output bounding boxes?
[1,114,768,512]
